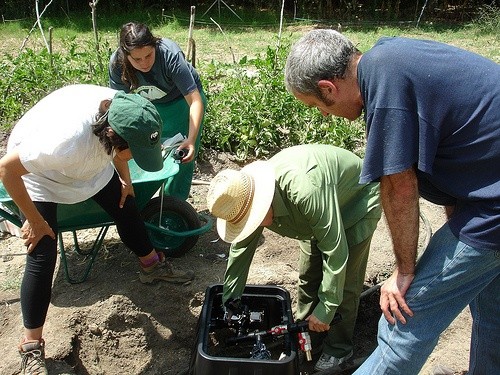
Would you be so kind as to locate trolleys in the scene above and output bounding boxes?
[1,144,213,284]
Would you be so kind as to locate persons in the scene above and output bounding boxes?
[283,29,500,375]
[107,22,208,206]
[0,84,195,375]
[206,143,383,375]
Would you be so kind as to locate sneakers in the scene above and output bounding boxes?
[18,338,48,375]
[138,251,196,286]
[314,350,353,371]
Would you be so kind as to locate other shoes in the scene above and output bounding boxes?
[432,366,469,375]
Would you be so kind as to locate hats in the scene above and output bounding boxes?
[206,160,275,244]
[107,90,164,173]
